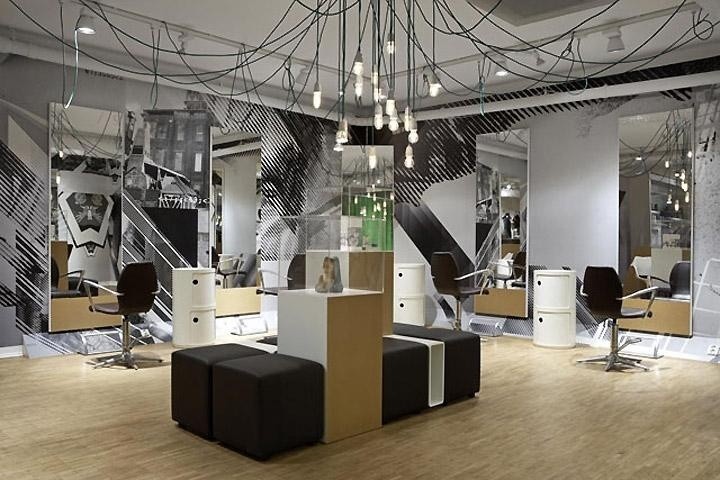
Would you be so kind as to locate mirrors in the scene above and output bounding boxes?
[47,100,128,334]
[616,106,696,339]
[208,123,264,319]
[474,128,530,320]
[339,142,396,251]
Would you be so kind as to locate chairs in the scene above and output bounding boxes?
[486,249,528,287]
[254,253,306,347]
[429,251,493,334]
[574,265,664,376]
[83,259,161,373]
[51,256,85,298]
[667,260,692,301]
[211,246,256,289]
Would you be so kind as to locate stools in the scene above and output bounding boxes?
[379,334,426,425]
[170,340,271,445]
[210,351,327,464]
[393,322,484,402]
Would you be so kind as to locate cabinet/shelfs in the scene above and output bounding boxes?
[532,268,579,348]
[170,265,215,347]
[393,263,428,326]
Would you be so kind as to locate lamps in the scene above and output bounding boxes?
[603,26,627,54]
[74,6,101,37]
[634,144,693,211]
[310,1,441,172]
[493,55,509,77]
[293,63,312,88]
[343,150,394,221]
